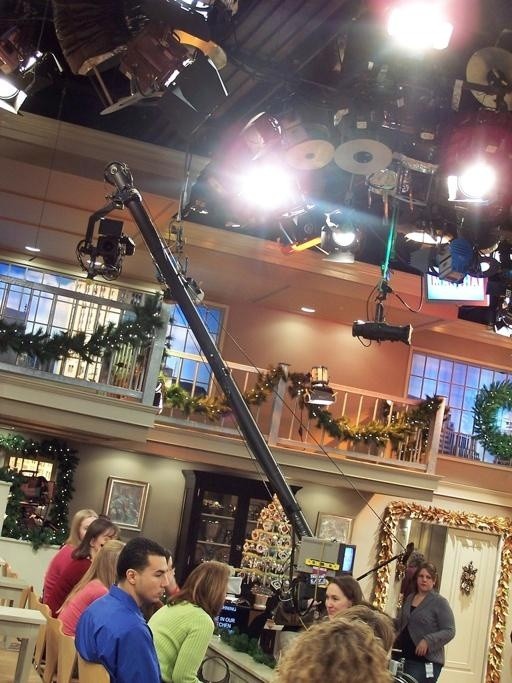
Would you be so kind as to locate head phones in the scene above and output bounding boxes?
[195,649,232,678]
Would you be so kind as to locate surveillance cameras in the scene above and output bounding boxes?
[302,383,339,410]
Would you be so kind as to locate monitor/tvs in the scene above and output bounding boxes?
[336,544,356,575]
[199,602,249,637]
[425,260,486,301]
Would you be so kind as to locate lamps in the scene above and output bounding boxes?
[305,364,337,408]
[1,20,50,117]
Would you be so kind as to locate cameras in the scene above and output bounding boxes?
[76,218,135,280]
[352,321,414,347]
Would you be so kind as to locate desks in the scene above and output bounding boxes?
[0,605,45,681]
[1,575,28,610]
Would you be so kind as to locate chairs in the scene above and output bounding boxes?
[76,650,112,683]
[45,616,78,681]
[19,586,31,609]
[27,590,53,671]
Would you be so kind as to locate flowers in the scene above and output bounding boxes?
[461,559,477,593]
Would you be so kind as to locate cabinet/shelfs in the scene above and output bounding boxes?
[174,468,304,590]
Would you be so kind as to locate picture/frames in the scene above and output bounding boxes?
[99,476,150,532]
[315,510,356,544]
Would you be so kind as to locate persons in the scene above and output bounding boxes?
[323,575,383,621]
[390,561,456,682]
[75,536,170,682]
[45,510,99,618]
[147,560,231,683]
[57,541,126,636]
[45,518,119,617]
